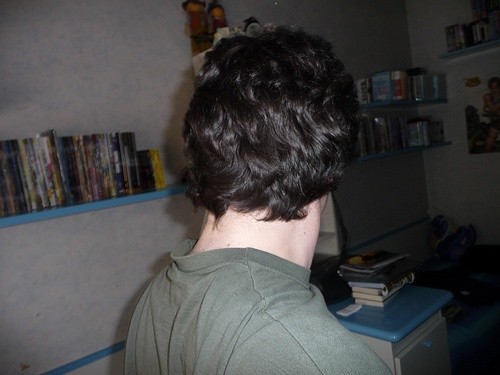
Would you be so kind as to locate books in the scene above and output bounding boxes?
[340,250,415,312]
[356,112,445,157]
[445,9,500,53]
[0,128,168,218]
[354,68,442,105]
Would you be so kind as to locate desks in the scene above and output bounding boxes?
[327,286,453,375]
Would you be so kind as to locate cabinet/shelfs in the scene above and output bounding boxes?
[341,67,452,161]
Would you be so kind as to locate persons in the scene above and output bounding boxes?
[123,24,394,375]
